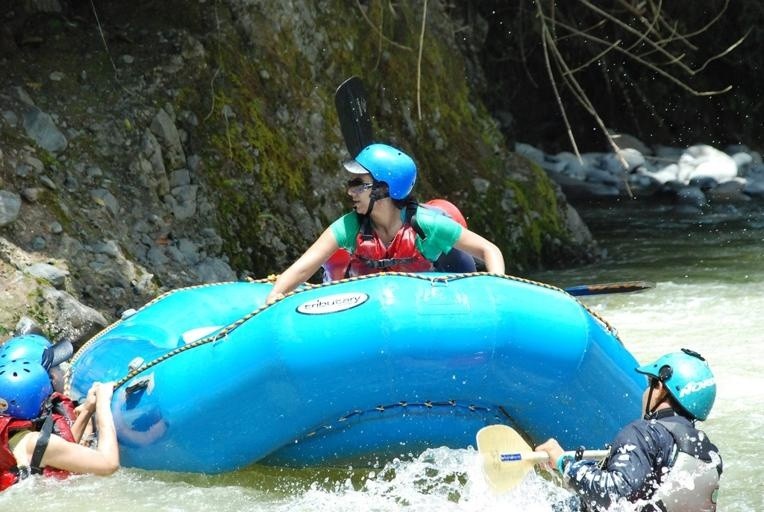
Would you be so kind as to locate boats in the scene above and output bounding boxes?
[59,270,676,472]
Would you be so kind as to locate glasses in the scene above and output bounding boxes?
[347,179,373,192]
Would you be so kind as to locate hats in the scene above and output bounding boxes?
[343,159,370,174]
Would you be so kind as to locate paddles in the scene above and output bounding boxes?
[336,73,375,157]
[562,279,651,294]
[476,424,610,490]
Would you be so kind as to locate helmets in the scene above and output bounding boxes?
[635,348,716,421]
[0,334,73,420]
[342,144,417,200]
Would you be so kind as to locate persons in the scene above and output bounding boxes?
[1,360,119,495]
[268,144,505,308]
[532,347,723,512]
[0,334,93,448]
